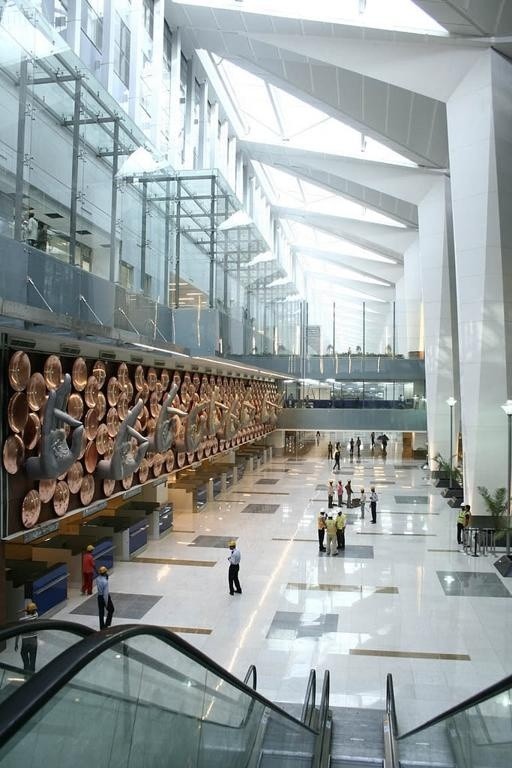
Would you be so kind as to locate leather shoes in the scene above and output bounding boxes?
[229,592,234,595]
[235,591,242,593]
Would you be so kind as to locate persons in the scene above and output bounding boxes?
[94,562,115,635]
[26,212,39,247]
[314,428,388,558]
[31,221,48,252]
[12,600,40,681]
[79,542,100,597]
[288,391,294,399]
[462,505,472,546]
[304,395,309,400]
[456,503,465,545]
[398,394,404,402]
[226,540,244,597]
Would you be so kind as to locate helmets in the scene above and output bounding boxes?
[329,480,333,483]
[348,479,351,482]
[328,513,333,517]
[320,508,326,512]
[27,603,37,609]
[336,509,341,512]
[361,487,364,490]
[98,567,107,573]
[370,486,375,489]
[229,542,236,546]
[87,545,95,551]
[338,480,342,482]
